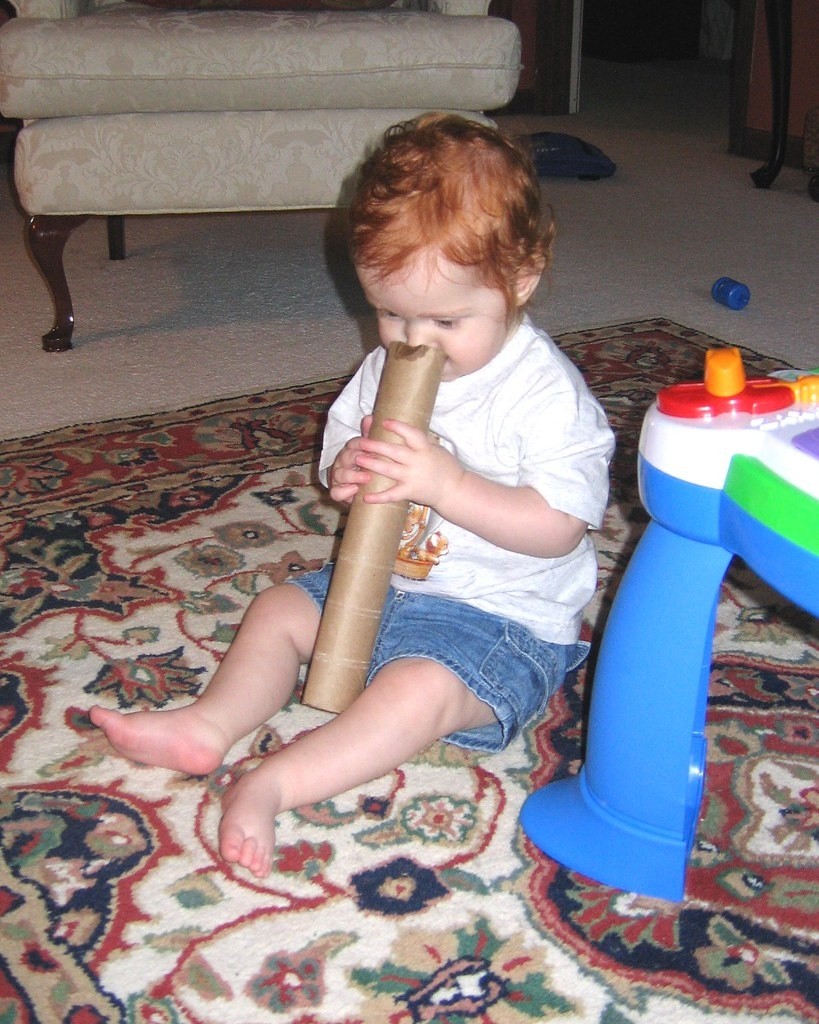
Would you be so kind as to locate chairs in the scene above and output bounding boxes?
[0,0,526,355]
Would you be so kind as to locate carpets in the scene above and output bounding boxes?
[0,313,819,1024]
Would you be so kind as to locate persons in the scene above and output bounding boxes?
[89,112,617,880]
